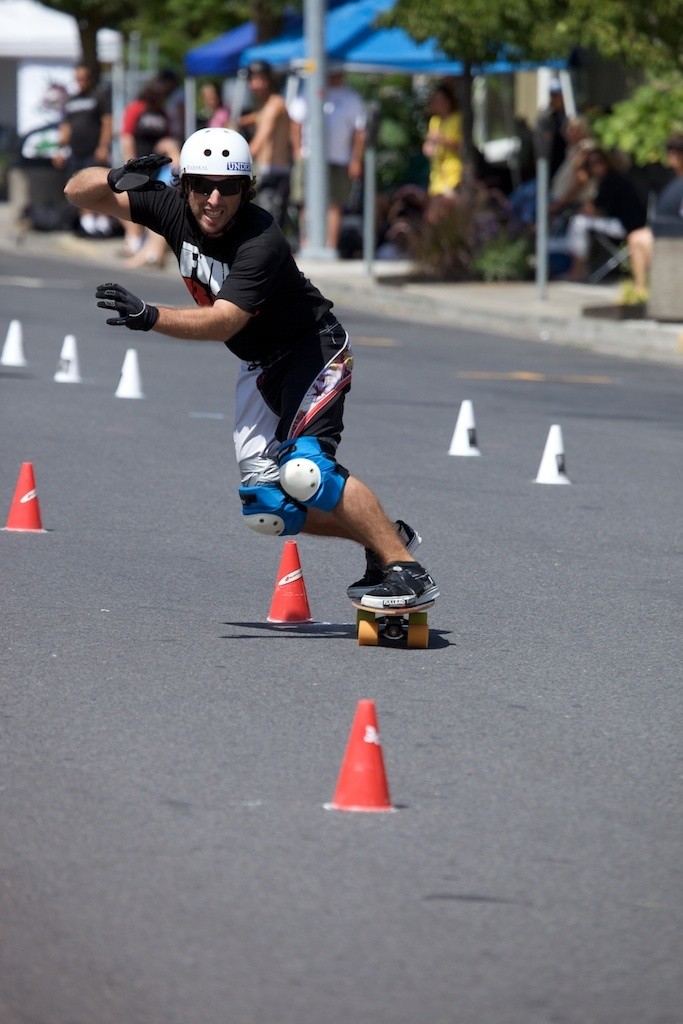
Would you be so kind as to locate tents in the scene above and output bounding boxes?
[183,10,303,140]
[231,1,579,288]
[1,0,122,169]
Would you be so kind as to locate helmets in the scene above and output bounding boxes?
[179,127,254,182]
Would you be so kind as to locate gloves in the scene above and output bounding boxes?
[95,282,159,331]
[107,154,173,194]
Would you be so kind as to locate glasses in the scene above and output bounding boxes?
[185,175,245,196]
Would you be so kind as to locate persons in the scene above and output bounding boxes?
[199,82,230,127]
[239,63,291,227]
[119,76,184,268]
[62,128,440,606]
[54,60,113,237]
[422,81,465,221]
[290,73,368,254]
[487,80,683,313]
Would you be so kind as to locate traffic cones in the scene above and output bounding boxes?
[530,424,572,485]
[0,458,51,535]
[265,539,315,624]
[0,317,30,369]
[321,697,403,814]
[447,398,482,458]
[52,333,81,385]
[114,348,146,401]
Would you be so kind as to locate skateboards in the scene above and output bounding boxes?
[345,579,436,650]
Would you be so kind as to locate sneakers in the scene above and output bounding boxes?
[347,519,422,599]
[360,561,441,610]
